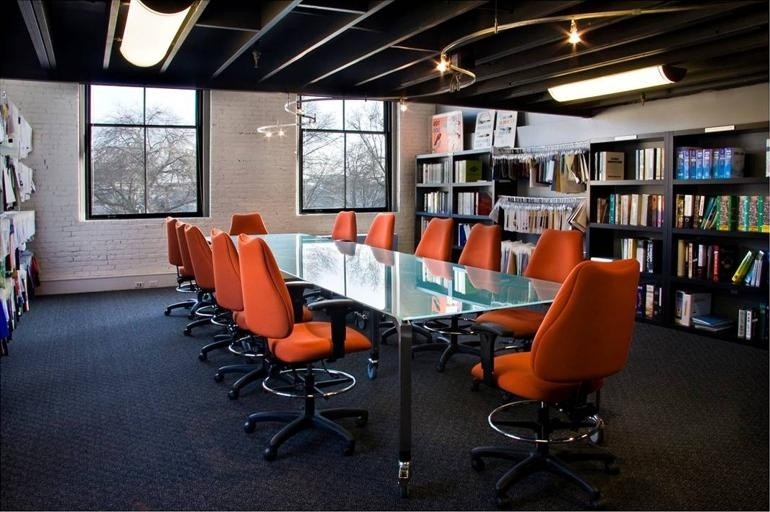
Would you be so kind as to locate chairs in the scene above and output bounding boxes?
[411,223,502,373]
[379,215,454,345]
[210,227,315,401]
[364,213,396,249]
[164,215,200,319]
[304,210,358,300]
[229,212,268,236]
[174,219,220,335]
[238,232,372,462]
[470,229,584,404]
[470,257,641,503]
[184,223,239,360]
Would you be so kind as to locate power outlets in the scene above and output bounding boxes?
[134,281,145,289]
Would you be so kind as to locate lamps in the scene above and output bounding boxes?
[119,0,196,68]
[547,64,689,103]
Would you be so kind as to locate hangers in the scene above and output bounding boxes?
[492,139,590,161]
[496,194,579,213]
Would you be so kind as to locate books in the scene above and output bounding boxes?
[636,284,663,319]
[675,290,733,332]
[421,216,430,240]
[590,237,663,274]
[677,239,729,282]
[594,150,625,181]
[457,191,493,216]
[422,160,451,185]
[765,137,770,178]
[675,146,746,181]
[423,191,449,214]
[737,303,768,340]
[457,222,473,247]
[454,159,483,184]
[675,193,770,233]
[731,248,765,288]
[635,147,666,181]
[596,192,665,229]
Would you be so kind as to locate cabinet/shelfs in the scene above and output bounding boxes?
[413,147,519,264]
[668,120,770,352]
[585,130,668,328]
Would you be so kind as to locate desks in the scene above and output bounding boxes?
[203,232,563,499]
